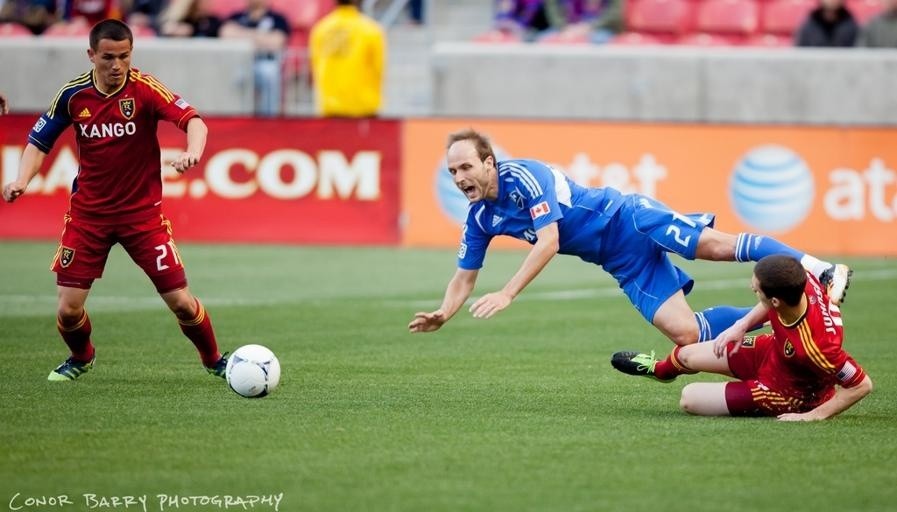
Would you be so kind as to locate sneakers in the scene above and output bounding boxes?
[48,346,96,381]
[612,349,660,376]
[202,350,230,378]
[818,262,853,307]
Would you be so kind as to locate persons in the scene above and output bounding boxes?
[0,0,72,36]
[848,1,895,50]
[792,1,858,49]
[532,1,626,47]
[61,1,130,36]
[0,15,233,385]
[606,253,873,422]
[304,0,387,119]
[404,126,854,349]
[493,1,550,45]
[405,0,426,34]
[122,1,199,38]
[215,0,292,115]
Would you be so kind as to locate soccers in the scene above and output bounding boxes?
[226,344,280,398]
